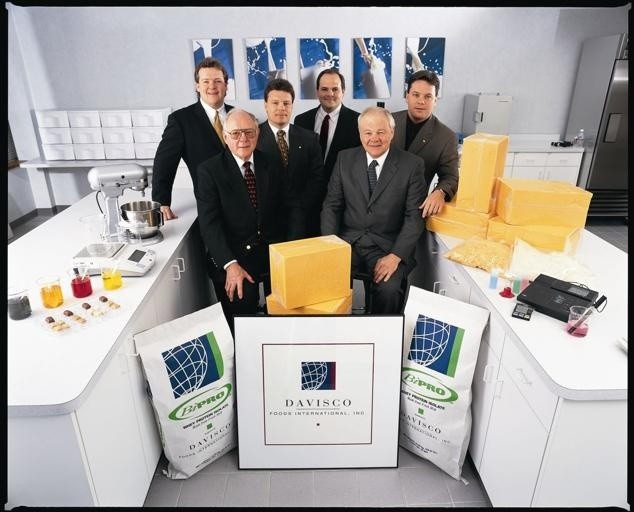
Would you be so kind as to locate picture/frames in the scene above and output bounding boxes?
[232,314,405,470]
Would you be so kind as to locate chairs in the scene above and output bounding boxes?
[351,245,418,315]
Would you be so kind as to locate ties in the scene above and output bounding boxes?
[213,111,226,148]
[320,115,330,163]
[277,129,291,165]
[367,160,379,200]
[243,162,258,207]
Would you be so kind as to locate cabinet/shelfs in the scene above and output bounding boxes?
[417,227,470,305]
[504,152,584,187]
[153,218,217,326]
[8,294,163,507]
[469,283,629,509]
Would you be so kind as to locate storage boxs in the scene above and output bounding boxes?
[34,107,172,160]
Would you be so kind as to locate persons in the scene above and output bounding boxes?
[254,79,324,239]
[389,70,459,217]
[197,109,296,336]
[321,107,427,316]
[294,69,364,209]
[152,58,237,219]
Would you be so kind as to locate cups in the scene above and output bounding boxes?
[100,259,124,290]
[567,305,592,338]
[36,273,64,308]
[7,286,32,320]
[68,266,92,297]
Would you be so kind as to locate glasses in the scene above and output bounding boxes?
[226,129,257,139]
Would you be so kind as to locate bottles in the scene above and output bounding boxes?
[576,129,584,150]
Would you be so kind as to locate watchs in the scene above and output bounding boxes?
[439,188,448,196]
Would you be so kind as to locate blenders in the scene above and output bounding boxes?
[87,163,164,247]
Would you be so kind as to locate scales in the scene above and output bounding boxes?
[72,241,157,277]
